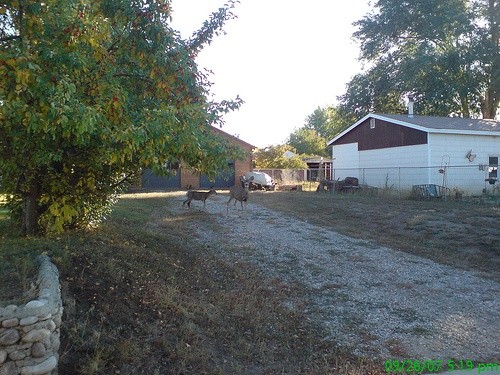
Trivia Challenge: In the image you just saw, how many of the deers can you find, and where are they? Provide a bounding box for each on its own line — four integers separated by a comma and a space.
182, 185, 218, 208
226, 175, 254, 217
